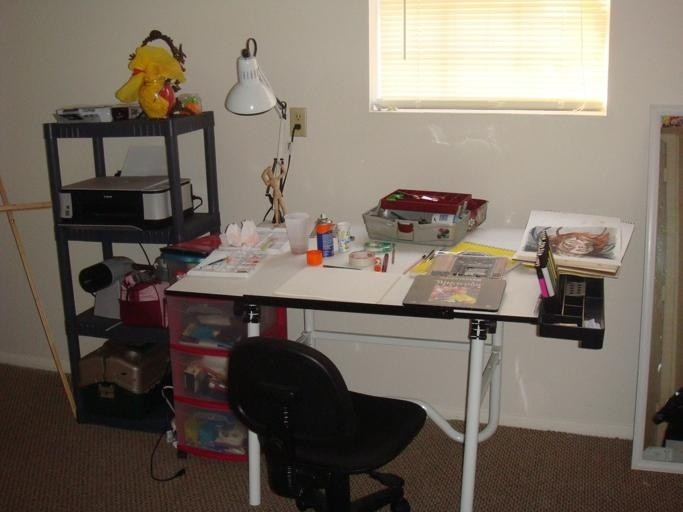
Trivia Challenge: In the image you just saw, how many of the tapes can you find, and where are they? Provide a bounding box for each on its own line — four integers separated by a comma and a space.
349, 250, 375, 268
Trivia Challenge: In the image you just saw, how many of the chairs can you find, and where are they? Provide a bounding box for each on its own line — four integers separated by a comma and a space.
228, 337, 426, 512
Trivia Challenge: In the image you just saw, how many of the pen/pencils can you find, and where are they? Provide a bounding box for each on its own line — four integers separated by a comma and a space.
199, 256, 229, 269
322, 264, 362, 272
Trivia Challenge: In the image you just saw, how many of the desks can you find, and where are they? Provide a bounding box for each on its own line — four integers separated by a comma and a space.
164, 220, 553, 511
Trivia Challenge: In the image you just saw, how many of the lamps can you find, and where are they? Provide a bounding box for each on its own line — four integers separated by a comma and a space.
222, 36, 288, 225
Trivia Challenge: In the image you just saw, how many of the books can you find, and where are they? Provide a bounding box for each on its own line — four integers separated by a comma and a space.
188, 250, 272, 277
514, 210, 634, 279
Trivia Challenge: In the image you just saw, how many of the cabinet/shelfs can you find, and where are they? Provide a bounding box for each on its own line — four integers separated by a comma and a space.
44, 108, 222, 434
166, 295, 288, 462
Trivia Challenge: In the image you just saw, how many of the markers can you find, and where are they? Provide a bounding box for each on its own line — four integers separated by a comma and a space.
375, 256, 381, 271
382, 254, 389, 272
534, 231, 557, 299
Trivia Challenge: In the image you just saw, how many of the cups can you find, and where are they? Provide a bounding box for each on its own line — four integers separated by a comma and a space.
285, 212, 310, 254
336, 222, 351, 253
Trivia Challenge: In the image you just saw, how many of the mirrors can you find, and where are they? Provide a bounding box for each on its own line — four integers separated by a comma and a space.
632, 107, 682, 477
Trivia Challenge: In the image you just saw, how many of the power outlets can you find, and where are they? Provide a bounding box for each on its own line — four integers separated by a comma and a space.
288, 106, 308, 139
